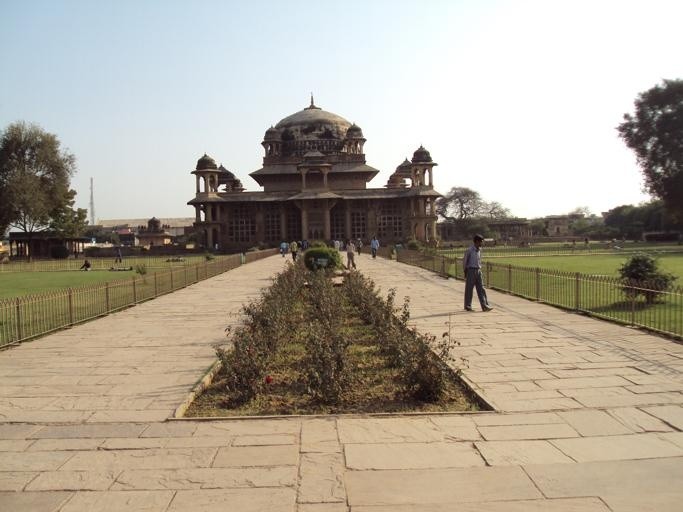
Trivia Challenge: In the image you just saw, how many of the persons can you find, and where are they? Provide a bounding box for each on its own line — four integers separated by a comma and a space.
371, 236, 379, 258
463, 233, 493, 312
346, 241, 356, 270
332, 238, 363, 255
80, 260, 92, 271
281, 239, 309, 262
115, 246, 122, 263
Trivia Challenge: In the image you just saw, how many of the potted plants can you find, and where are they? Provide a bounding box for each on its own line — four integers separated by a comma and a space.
305, 247, 343, 271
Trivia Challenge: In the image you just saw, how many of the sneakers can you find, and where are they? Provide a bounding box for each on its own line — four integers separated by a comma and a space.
467, 305, 494, 311
